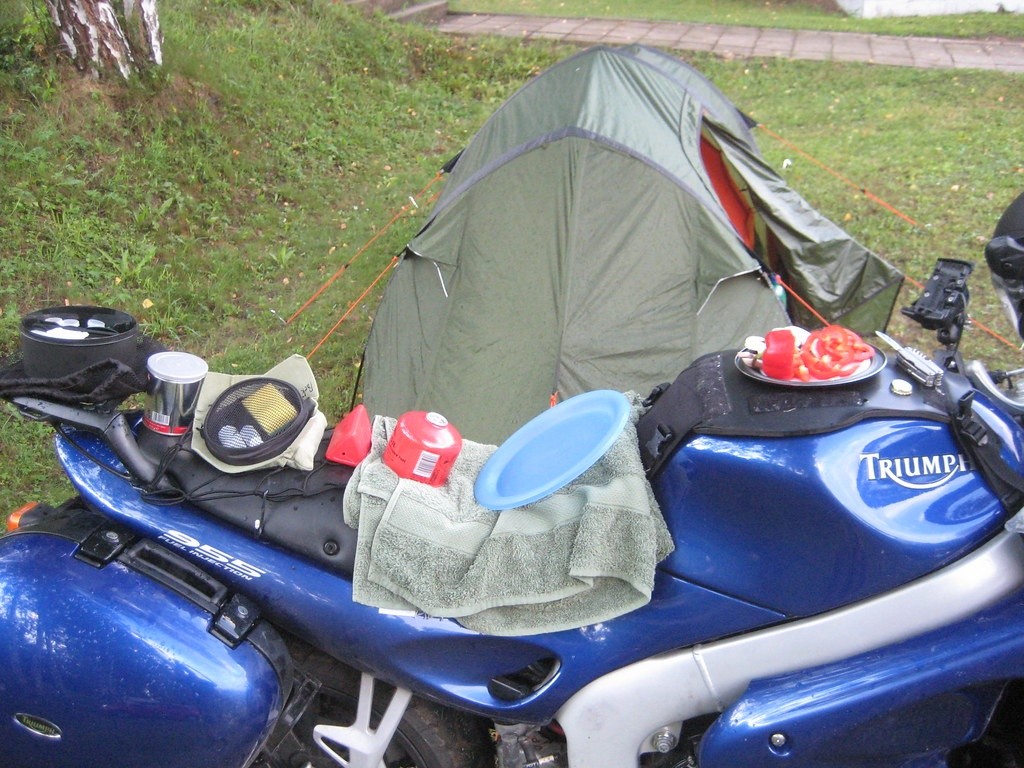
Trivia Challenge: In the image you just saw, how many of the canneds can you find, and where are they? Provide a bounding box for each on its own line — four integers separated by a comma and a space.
142, 352, 209, 436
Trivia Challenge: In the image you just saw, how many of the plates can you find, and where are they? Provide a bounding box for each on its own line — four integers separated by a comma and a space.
733, 343, 888, 387
473, 390, 630, 509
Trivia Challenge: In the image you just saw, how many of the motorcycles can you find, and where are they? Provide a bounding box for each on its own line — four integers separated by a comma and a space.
0, 302, 1024, 768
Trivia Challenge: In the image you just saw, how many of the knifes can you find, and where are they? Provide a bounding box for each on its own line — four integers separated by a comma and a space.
875, 330, 943, 387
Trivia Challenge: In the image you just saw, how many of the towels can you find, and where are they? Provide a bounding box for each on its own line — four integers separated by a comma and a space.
342, 388, 677, 638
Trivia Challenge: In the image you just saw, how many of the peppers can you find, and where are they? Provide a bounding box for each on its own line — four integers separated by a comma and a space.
750, 324, 875, 383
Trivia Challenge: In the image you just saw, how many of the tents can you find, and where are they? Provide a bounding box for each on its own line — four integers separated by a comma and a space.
361, 42, 905, 446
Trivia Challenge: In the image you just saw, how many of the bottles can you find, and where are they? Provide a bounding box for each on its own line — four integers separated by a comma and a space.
384, 411, 463, 487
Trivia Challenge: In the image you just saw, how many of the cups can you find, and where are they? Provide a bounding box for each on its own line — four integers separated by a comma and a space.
141, 350, 209, 437
